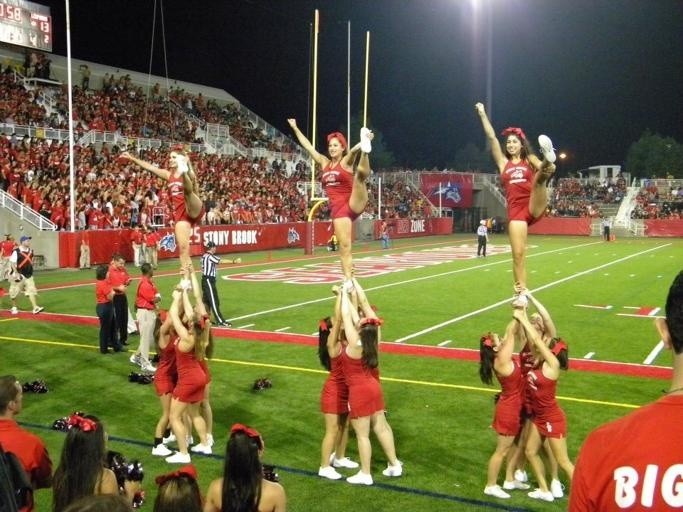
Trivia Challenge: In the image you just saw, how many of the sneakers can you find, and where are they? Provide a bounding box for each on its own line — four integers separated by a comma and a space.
152, 430, 214, 463
484, 469, 563, 502
176, 155, 188, 172
360, 127, 372, 153
101, 340, 129, 352
179, 279, 193, 289
218, 320, 232, 327
318, 453, 401, 485
538, 134, 557, 162
341, 279, 353, 291
11, 307, 18, 314
129, 354, 159, 373
512, 293, 528, 307
32, 305, 44, 314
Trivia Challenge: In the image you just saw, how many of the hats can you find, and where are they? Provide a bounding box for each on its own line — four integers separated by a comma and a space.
20, 235, 32, 242
206, 241, 216, 248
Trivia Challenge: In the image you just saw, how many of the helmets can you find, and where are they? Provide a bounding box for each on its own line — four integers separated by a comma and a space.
480, 220, 487, 225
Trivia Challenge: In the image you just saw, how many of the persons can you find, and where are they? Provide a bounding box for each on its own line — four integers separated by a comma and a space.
0, 53, 330, 226
377, 221, 391, 249
95, 255, 161, 372
479, 281, 576, 501
2, 233, 44, 315
474, 102, 555, 308
151, 264, 214, 464
319, 262, 402, 485
80, 225, 91, 270
288, 118, 374, 292
546, 170, 626, 218
120, 143, 205, 291
200, 242, 241, 327
568, 271, 683, 511
1, 373, 287, 511
600, 217, 615, 241
631, 179, 682, 219
364, 179, 434, 221
